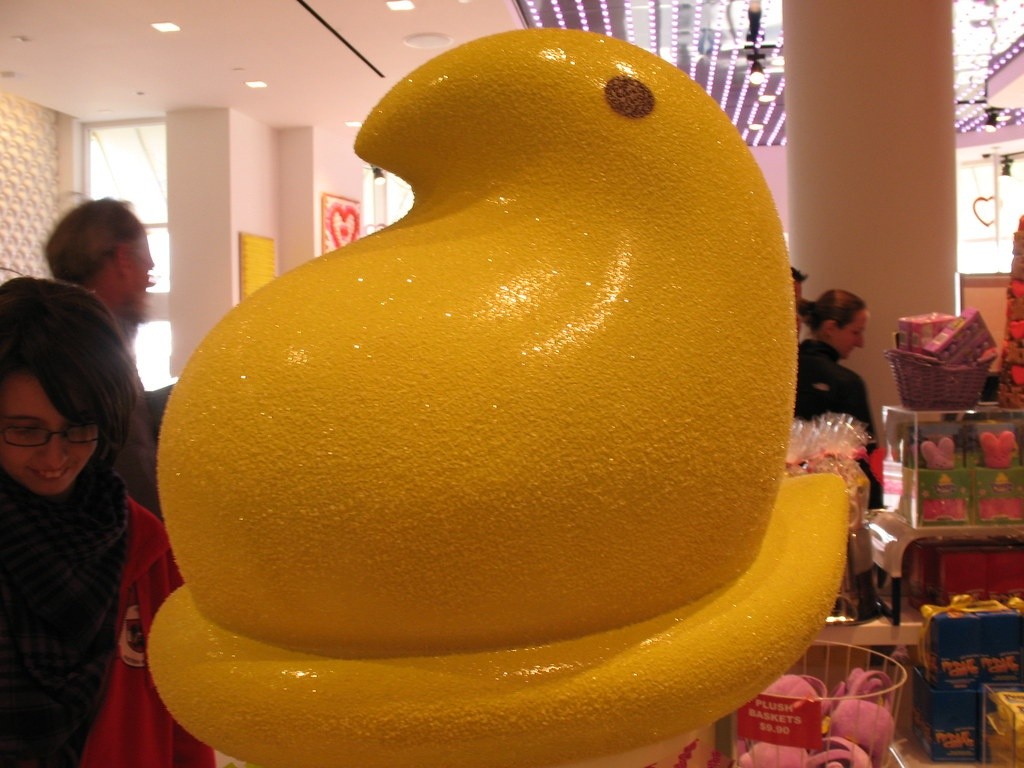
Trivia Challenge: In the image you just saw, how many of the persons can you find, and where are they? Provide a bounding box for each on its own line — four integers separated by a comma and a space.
45, 199, 174, 515
0, 276, 216, 768
796, 289, 883, 509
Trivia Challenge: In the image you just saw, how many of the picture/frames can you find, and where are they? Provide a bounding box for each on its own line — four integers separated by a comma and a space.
319, 193, 361, 257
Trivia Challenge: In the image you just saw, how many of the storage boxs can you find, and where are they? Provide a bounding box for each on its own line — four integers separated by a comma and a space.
882, 307, 1024, 768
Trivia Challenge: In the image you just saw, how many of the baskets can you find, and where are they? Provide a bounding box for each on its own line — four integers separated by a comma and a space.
884, 346, 994, 410
734, 638, 907, 767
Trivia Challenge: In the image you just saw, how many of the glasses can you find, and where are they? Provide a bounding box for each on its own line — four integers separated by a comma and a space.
0, 417, 99, 447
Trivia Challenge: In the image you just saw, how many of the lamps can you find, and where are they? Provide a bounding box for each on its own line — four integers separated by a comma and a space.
747, 55, 767, 85
1001, 159, 1013, 178
984, 110, 998, 133
371, 166, 384, 184
747, 0, 762, 36
